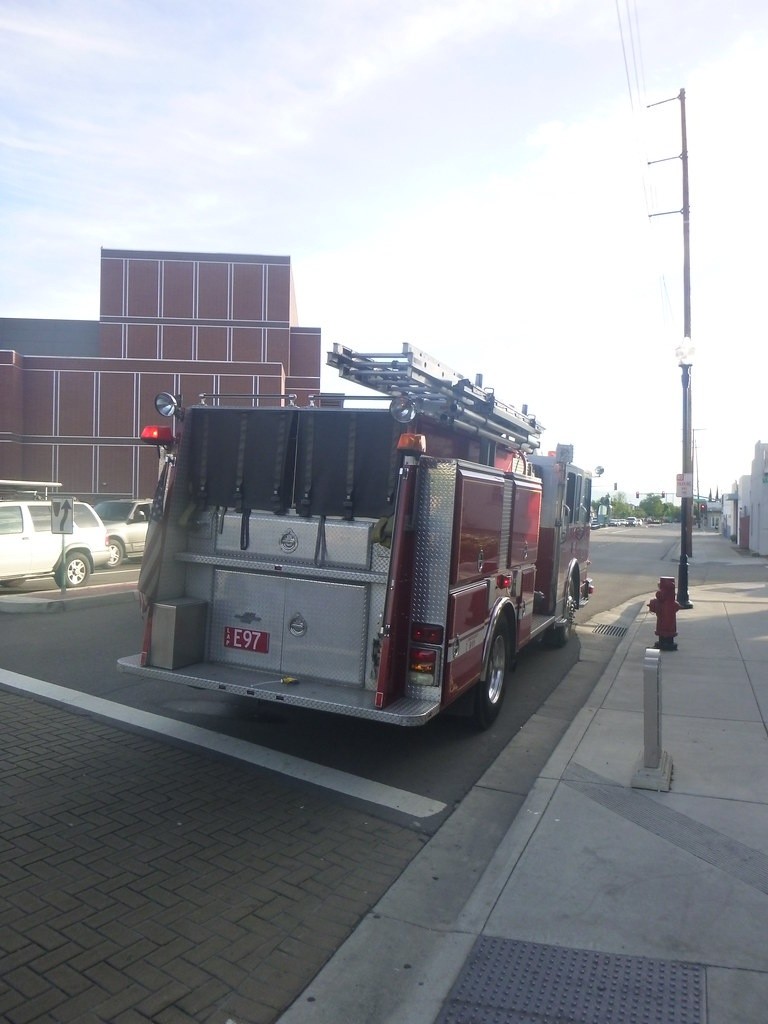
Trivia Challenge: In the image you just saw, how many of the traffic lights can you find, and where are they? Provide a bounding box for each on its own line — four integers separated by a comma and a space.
662, 491, 665, 498
636, 491, 639, 498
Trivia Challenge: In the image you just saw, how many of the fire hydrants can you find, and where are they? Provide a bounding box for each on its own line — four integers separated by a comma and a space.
645, 577, 683, 652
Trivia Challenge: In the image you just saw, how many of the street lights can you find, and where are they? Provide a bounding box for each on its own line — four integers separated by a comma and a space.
675, 333, 696, 610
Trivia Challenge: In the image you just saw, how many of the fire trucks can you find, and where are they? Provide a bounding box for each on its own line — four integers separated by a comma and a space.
136, 340, 594, 733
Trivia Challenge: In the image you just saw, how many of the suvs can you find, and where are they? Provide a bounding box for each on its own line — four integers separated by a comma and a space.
0, 479, 112, 589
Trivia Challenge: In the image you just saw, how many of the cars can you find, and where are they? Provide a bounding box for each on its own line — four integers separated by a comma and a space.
91, 498, 152, 571
591, 517, 643, 530
